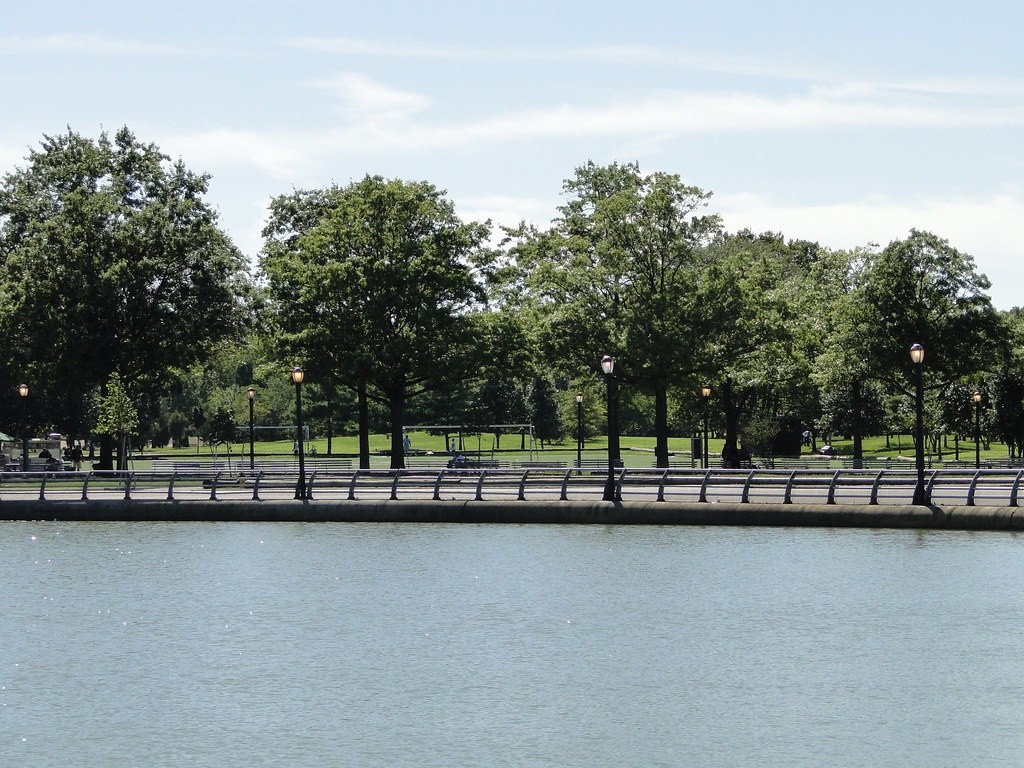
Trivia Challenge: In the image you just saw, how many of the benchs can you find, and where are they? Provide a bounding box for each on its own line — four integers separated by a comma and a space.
152, 460, 225, 482
842, 457, 1024, 469
708, 460, 831, 469
652, 461, 698, 469
29, 463, 71, 476
511, 461, 568, 476
573, 459, 624, 475
235, 460, 510, 480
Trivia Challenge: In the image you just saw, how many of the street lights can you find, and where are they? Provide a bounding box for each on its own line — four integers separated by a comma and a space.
910, 344, 926, 505
576, 393, 584, 476
245, 388, 255, 476
19, 384, 29, 471
601, 355, 614, 501
702, 385, 711, 468
973, 392, 981, 467
290, 368, 305, 499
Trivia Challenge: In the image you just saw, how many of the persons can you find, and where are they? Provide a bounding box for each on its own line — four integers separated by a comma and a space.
403, 435, 411, 455
70, 445, 84, 471
451, 439, 456, 452
292, 438, 299, 456
39, 448, 59, 470
310, 445, 317, 458
